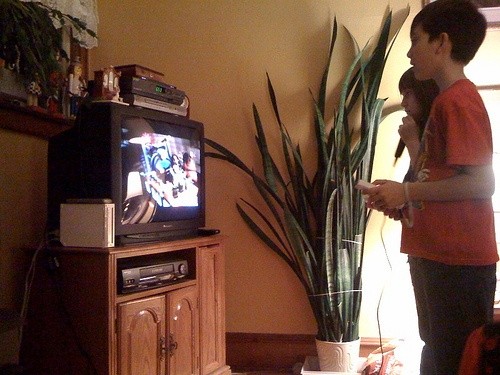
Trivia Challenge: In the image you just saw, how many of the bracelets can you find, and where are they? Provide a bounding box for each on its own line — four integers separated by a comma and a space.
403, 182, 411, 203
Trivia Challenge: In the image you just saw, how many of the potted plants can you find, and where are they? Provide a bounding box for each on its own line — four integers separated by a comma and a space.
204, 6, 408, 375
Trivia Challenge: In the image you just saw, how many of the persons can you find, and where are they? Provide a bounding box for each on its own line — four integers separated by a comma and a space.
135, 131, 197, 191
398, 66, 440, 184
362, 0, 499, 375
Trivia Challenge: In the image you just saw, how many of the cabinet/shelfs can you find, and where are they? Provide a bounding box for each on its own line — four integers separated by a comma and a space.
0, 1, 102, 137
18, 230, 232, 375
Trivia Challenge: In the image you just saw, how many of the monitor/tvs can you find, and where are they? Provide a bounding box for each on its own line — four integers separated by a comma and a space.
48, 101, 206, 246
